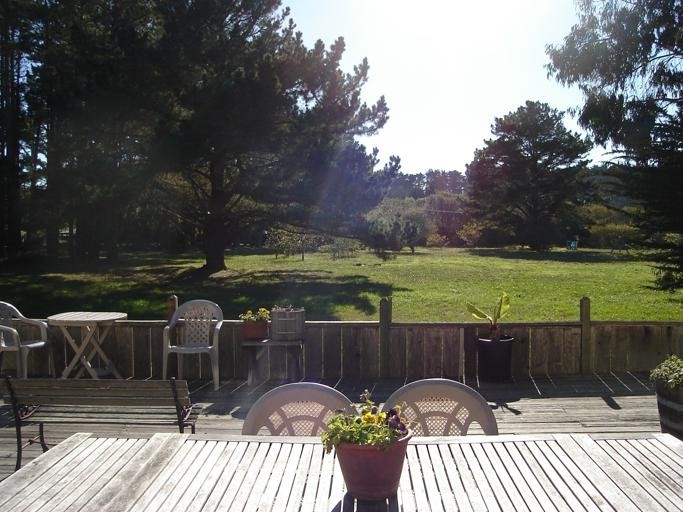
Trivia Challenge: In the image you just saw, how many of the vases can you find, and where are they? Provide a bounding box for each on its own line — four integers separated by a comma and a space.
330, 428, 413, 504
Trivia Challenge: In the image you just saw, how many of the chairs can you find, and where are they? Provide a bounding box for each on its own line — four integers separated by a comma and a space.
240, 381, 359, 440
374, 377, 500, 440
0, 299, 56, 381
160, 298, 224, 392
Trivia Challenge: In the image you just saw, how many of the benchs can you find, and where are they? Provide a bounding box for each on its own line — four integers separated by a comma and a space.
0, 373, 204, 477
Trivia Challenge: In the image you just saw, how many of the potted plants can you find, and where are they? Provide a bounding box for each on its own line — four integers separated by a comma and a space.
464, 289, 515, 384
237, 306, 271, 343
270, 305, 305, 342
645, 353, 683, 442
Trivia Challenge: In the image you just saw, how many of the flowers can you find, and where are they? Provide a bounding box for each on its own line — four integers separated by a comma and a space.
317, 386, 416, 461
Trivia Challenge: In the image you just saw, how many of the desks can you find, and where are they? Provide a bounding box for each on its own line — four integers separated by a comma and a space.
240, 338, 305, 387
45, 310, 129, 380
0, 429, 683, 512
